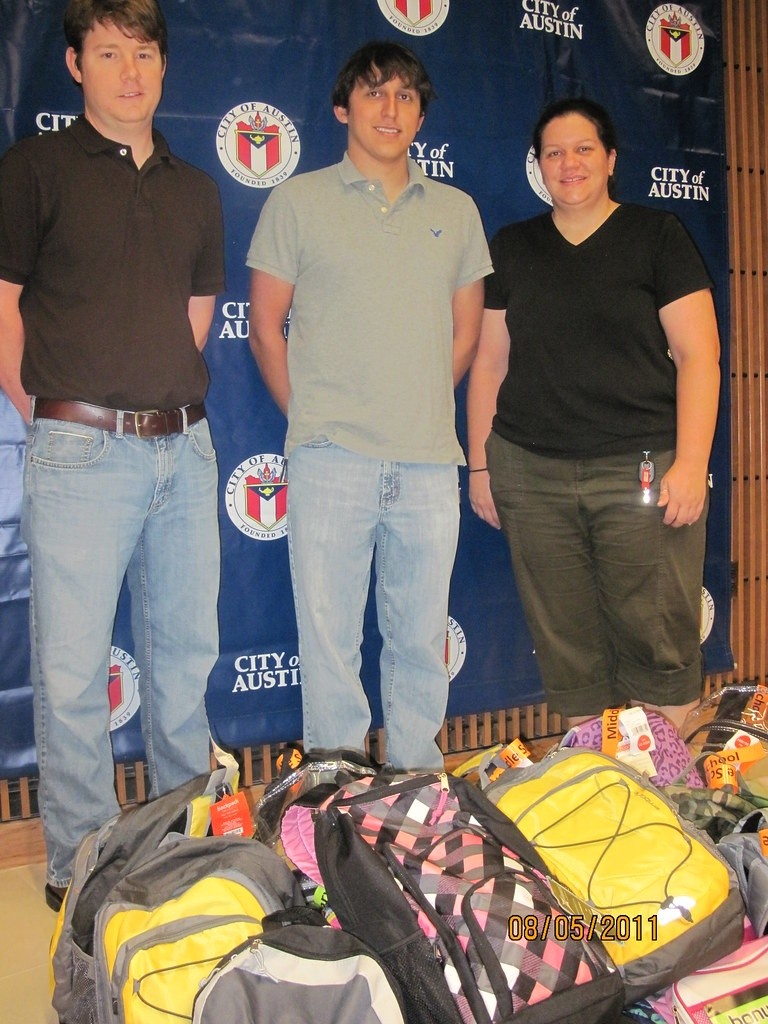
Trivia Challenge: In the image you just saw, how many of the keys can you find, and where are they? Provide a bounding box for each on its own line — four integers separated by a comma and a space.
638, 461, 654, 503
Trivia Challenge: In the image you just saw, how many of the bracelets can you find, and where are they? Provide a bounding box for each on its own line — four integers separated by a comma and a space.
469, 468, 488, 472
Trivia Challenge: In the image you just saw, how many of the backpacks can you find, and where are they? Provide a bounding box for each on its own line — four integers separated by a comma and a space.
47, 681, 768, 1024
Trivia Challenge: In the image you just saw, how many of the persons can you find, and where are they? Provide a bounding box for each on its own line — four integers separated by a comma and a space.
245, 40, 496, 772
0, 0, 225, 906
469, 98, 720, 737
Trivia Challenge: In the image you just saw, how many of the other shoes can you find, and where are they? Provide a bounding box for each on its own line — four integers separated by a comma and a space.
44, 884, 66, 908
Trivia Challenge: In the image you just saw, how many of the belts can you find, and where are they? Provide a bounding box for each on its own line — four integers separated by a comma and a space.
33, 399, 206, 439
